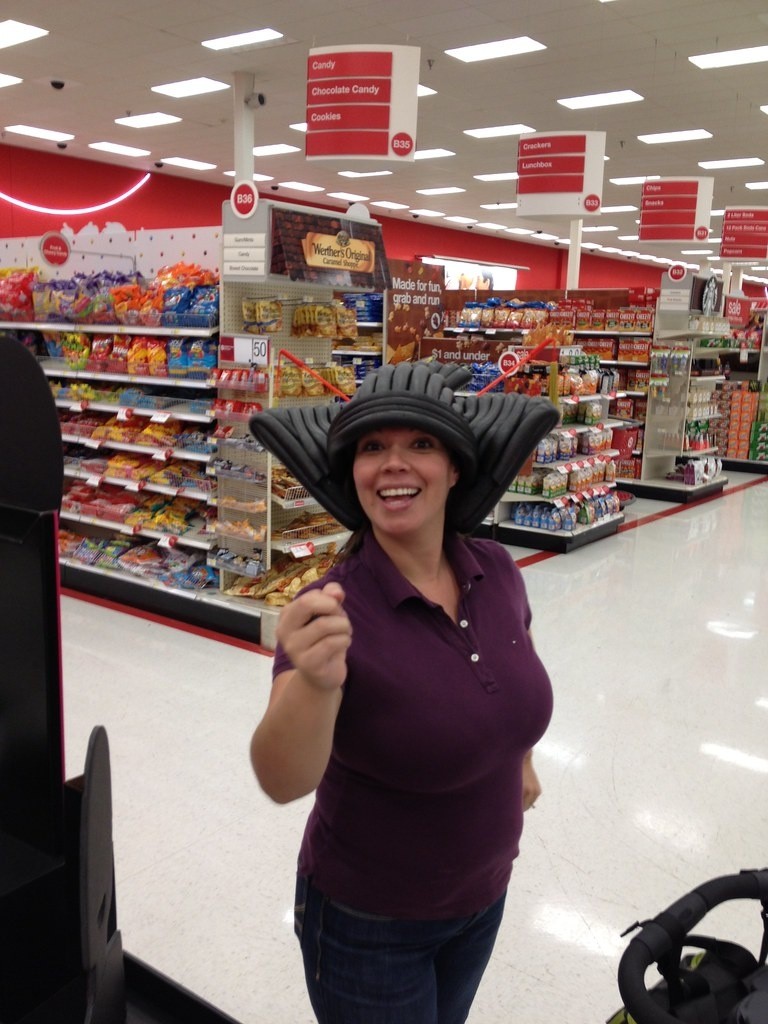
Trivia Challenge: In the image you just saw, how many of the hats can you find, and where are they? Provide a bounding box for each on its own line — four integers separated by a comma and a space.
252, 338, 559, 533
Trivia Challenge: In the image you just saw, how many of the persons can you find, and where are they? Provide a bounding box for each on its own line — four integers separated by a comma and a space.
251, 360, 555, 1024
456, 271, 494, 291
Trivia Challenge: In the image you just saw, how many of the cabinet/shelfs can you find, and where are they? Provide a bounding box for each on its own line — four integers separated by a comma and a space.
0, 200, 768, 648
